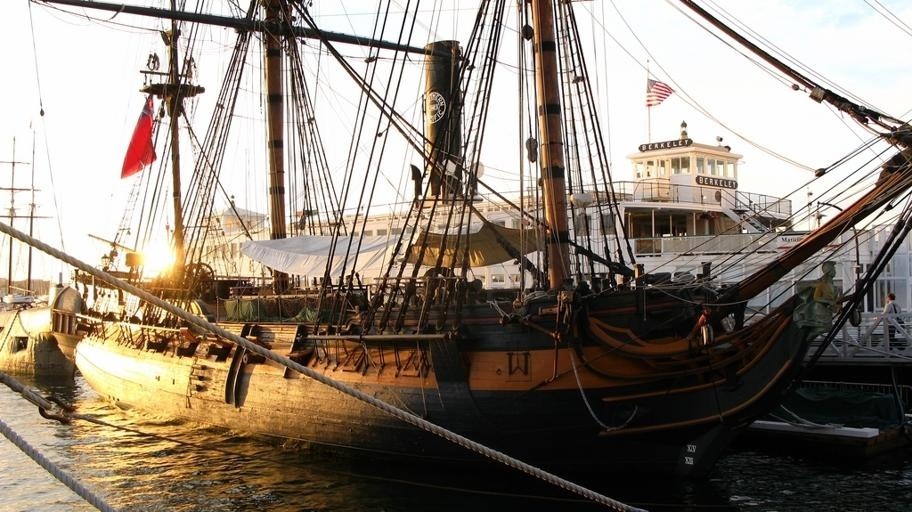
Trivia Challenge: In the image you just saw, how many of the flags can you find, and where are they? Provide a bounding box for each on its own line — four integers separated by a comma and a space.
119, 94, 156, 177
646, 79, 673, 106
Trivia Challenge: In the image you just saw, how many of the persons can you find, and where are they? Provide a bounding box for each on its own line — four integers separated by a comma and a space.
794, 261, 841, 339
881, 293, 901, 351
